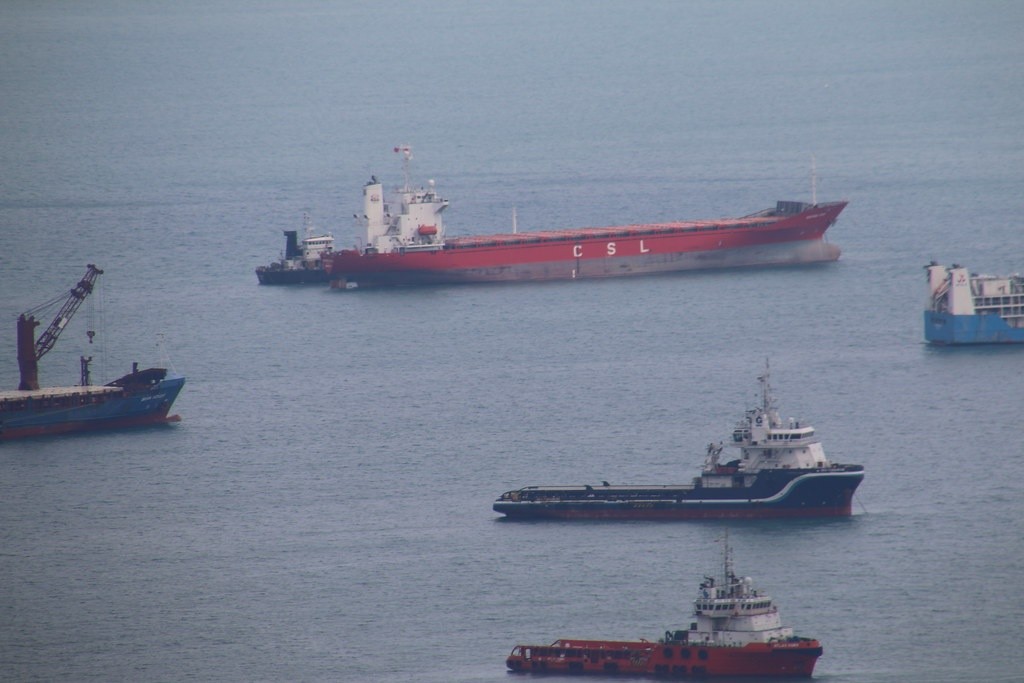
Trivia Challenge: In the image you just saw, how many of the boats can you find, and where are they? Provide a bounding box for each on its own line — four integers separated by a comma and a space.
320, 140, 849, 289
493, 357, 865, 518
255, 213, 341, 285
0, 263, 186, 443
506, 527, 823, 678
923, 260, 1024, 343
